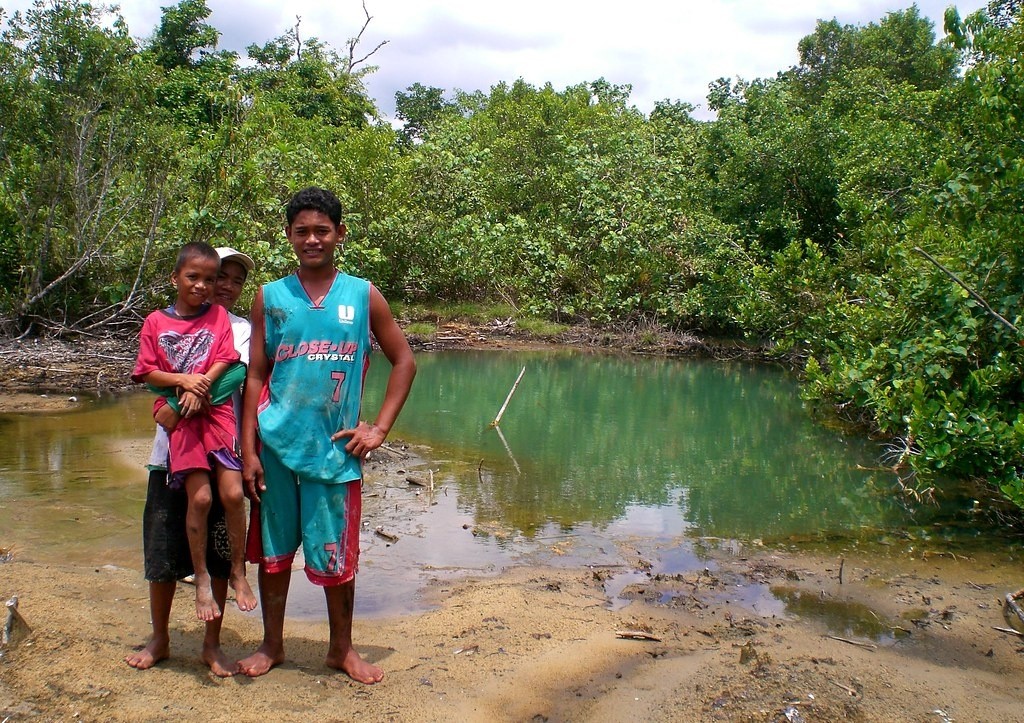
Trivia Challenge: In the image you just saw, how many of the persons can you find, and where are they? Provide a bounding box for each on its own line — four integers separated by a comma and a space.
239, 188, 417, 683
126, 247, 251, 676
131, 242, 258, 621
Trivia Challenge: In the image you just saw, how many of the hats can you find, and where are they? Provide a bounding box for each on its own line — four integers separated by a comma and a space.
214, 246, 255, 271
